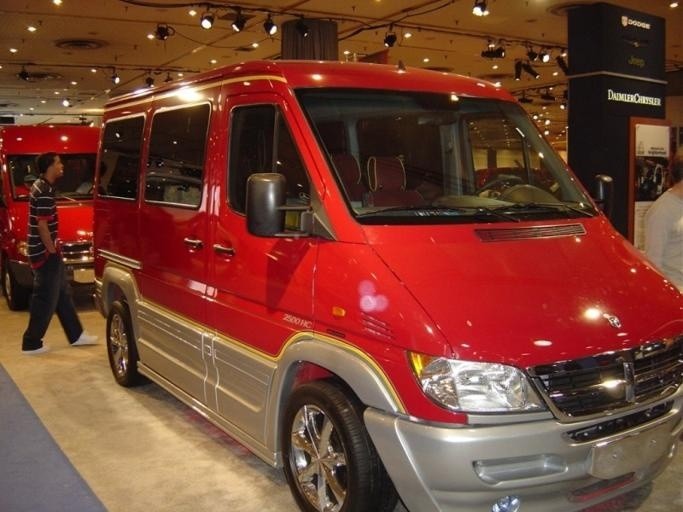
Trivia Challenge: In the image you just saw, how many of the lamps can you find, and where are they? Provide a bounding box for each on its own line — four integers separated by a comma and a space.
381, 23, 398, 48
471, 0, 488, 18
478, 39, 568, 107
198, 5, 279, 37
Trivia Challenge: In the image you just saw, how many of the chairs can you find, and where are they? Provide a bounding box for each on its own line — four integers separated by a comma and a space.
330, 150, 422, 211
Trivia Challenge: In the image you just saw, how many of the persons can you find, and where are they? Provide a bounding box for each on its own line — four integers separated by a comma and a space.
21, 152, 98, 353
642, 154, 683, 291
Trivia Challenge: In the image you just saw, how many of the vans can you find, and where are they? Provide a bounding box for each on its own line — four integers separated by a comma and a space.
90, 60, 681, 511
0, 123, 107, 312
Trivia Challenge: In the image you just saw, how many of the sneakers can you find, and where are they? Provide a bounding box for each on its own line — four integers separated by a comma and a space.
72, 331, 101, 345
22, 348, 46, 355
